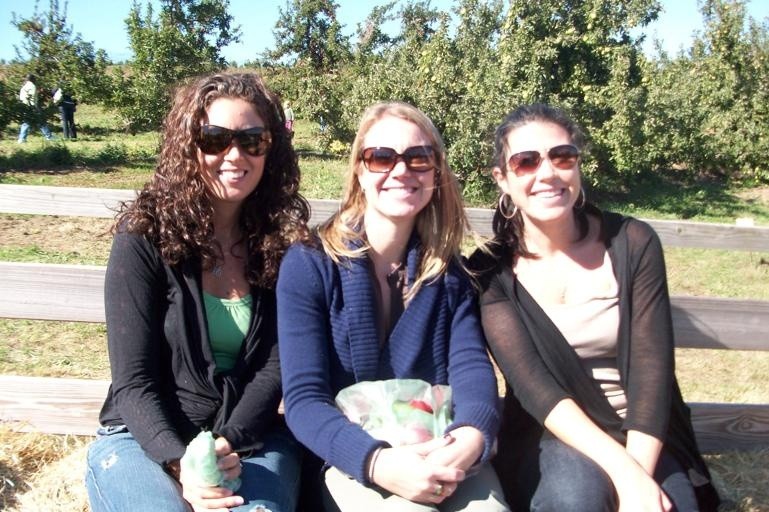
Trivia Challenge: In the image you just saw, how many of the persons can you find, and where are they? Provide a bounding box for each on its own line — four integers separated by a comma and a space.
16, 74, 59, 144
274, 98, 516, 511
82, 71, 315, 512
469, 103, 721, 511
49, 74, 78, 142
281, 101, 294, 132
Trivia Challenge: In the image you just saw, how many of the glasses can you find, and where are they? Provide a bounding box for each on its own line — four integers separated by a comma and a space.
358, 144, 439, 174
192, 123, 274, 158
506, 143, 579, 180
